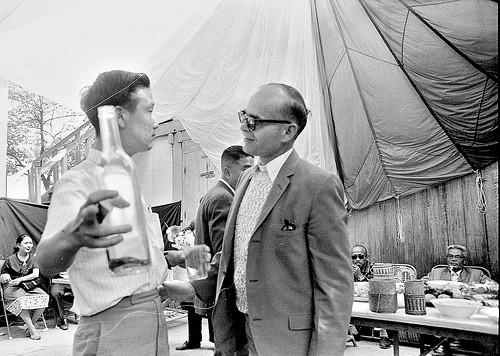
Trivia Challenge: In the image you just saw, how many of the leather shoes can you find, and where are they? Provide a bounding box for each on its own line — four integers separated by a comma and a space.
379, 336, 390, 349
175, 341, 201, 349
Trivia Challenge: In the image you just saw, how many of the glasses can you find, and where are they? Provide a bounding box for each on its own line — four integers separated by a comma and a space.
238, 110, 294, 131
280, 217, 296, 231
351, 254, 366, 260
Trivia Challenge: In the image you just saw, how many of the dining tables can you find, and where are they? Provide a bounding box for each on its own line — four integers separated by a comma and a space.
351, 293, 499, 356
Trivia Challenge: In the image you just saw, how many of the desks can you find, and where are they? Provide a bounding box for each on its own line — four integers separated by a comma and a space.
52, 278, 79, 325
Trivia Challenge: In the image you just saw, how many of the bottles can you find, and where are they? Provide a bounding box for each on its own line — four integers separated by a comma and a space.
96, 105, 151, 274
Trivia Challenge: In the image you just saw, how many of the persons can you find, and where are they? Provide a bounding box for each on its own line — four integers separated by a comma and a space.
194, 145, 254, 262
175, 197, 214, 350
158, 82, 354, 356
422, 245, 498, 286
0, 234, 49, 339
348, 245, 391, 349
32, 70, 211, 356
50, 274, 80, 330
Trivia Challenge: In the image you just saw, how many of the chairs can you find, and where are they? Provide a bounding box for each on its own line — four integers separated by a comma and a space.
371, 263, 417, 283
0, 259, 48, 340
431, 264, 491, 286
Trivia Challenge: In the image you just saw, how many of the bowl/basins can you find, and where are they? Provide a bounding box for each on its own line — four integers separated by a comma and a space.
432, 298, 483, 318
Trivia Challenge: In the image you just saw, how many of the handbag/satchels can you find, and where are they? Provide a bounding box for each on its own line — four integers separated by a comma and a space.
20, 277, 43, 292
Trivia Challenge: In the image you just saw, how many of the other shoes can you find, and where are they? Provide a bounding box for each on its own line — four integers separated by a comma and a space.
29, 332, 41, 340
26, 328, 30, 336
58, 321, 69, 330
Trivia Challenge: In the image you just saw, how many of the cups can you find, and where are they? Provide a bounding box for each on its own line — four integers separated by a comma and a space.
184, 246, 208, 280
